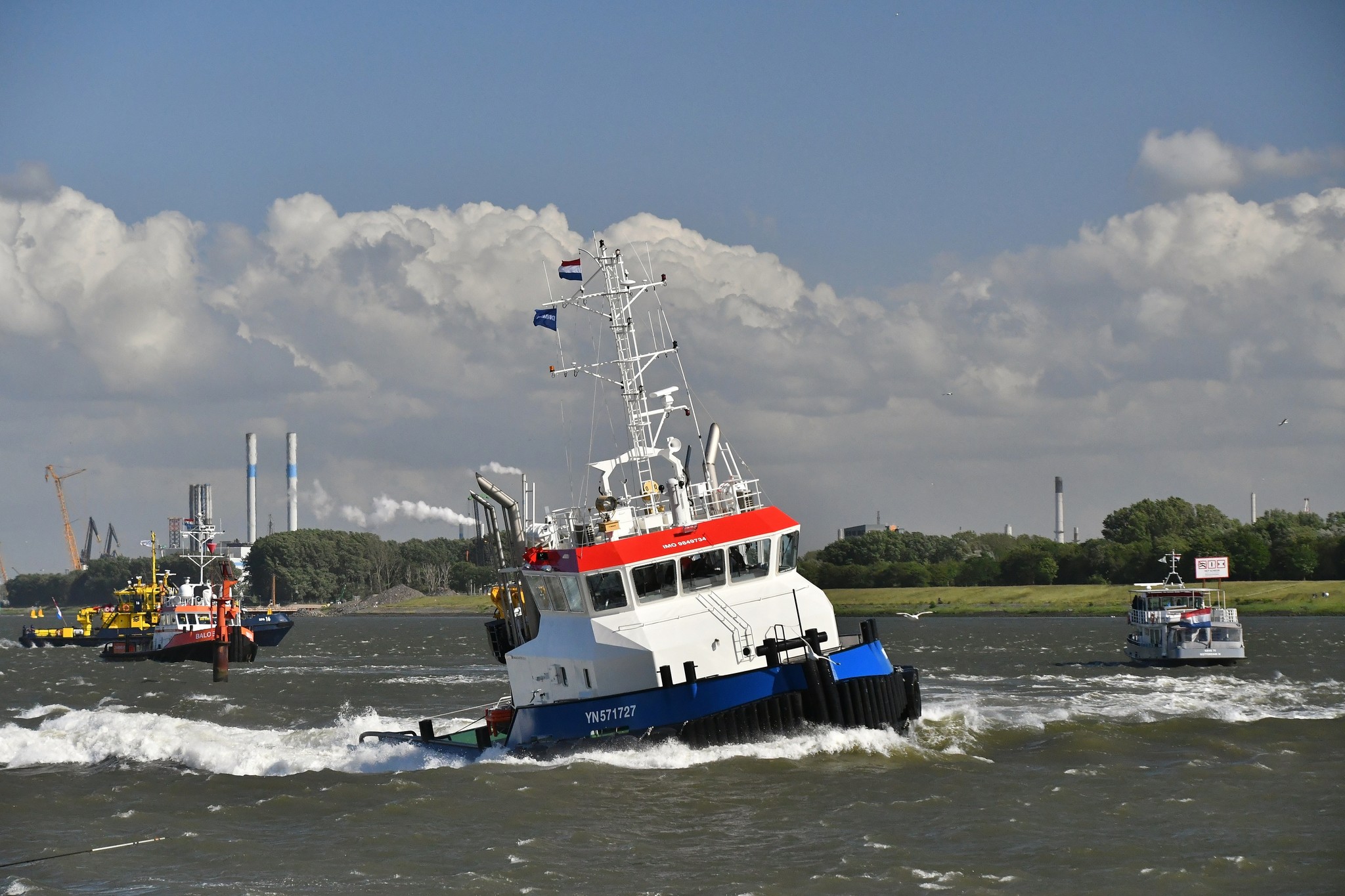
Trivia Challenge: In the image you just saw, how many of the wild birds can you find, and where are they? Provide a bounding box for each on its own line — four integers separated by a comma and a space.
1261, 478, 1265, 481
942, 392, 953, 396
895, 611, 934, 621
530, 688, 542, 703
931, 483, 934, 485
1278, 417, 1289, 427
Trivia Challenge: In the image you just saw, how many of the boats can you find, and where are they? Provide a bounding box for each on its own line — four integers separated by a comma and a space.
199, 612, 295, 646
1123, 548, 1248, 666
99, 543, 257, 664
18, 530, 179, 648
360, 232, 924, 766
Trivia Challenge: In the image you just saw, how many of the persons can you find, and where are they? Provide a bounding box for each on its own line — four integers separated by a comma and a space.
1176, 599, 1182, 606
180, 618, 185, 624
1131, 595, 1143, 622
665, 547, 746, 585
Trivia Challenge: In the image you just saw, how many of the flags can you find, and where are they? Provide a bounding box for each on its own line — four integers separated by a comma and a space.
558, 259, 582, 280
183, 519, 194, 527
1179, 607, 1212, 627
533, 308, 557, 332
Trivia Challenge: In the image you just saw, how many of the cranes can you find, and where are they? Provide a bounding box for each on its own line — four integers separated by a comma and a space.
103, 523, 121, 556
44, 465, 88, 571
80, 516, 101, 567
0, 557, 8, 582
